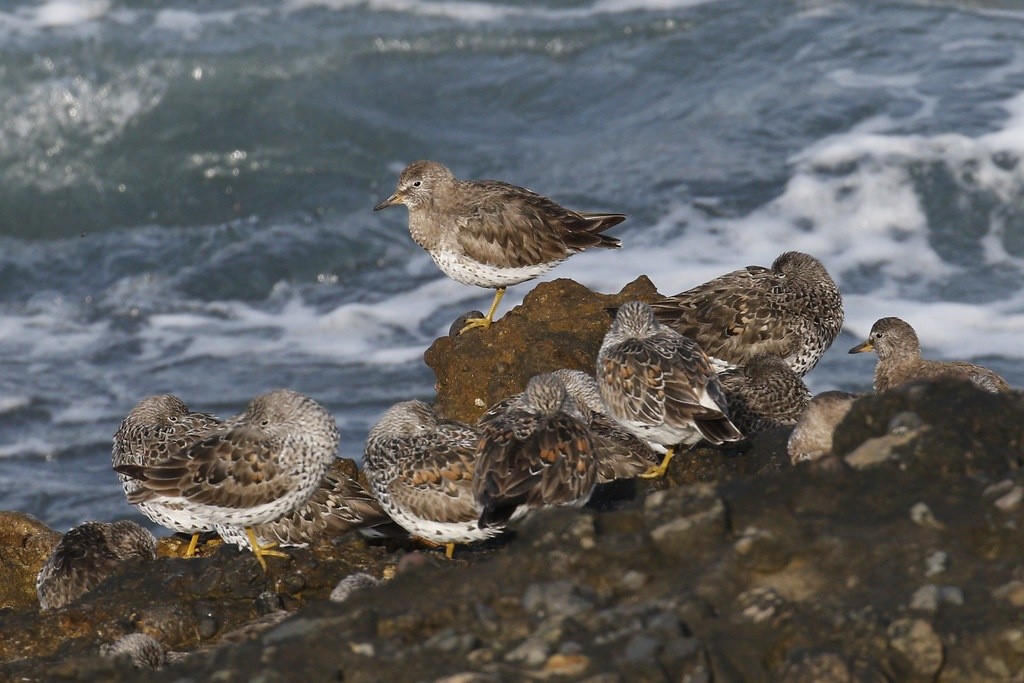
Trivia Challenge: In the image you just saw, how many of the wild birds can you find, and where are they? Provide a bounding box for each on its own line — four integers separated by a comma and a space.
330, 572, 379, 604
648, 250, 844, 378
112, 389, 340, 569
107, 633, 191, 674
597, 300, 742, 480
363, 399, 506, 582
373, 160, 630, 339
716, 353, 814, 432
786, 390, 867, 466
36, 520, 159, 609
472, 368, 660, 530
847, 317, 1013, 396
